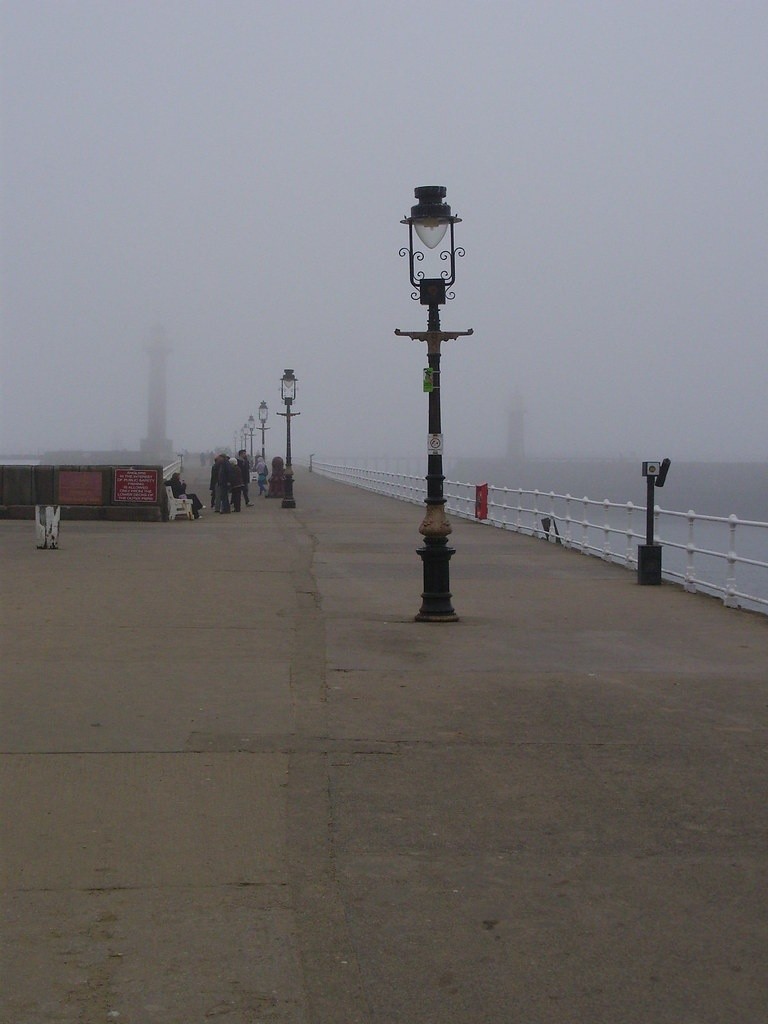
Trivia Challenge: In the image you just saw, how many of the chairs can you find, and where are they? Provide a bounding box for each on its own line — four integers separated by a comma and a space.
249, 471, 258, 482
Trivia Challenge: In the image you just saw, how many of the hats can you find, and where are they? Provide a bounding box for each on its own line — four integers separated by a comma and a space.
229, 457, 238, 465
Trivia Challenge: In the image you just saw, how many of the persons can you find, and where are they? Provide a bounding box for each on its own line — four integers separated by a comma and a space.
256, 457, 268, 496
171, 473, 206, 519
209, 450, 254, 514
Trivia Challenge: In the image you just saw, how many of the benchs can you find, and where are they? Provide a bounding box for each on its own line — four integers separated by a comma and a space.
165, 485, 196, 522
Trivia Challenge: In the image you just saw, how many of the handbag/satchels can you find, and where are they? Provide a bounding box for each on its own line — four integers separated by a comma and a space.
264, 465, 268, 476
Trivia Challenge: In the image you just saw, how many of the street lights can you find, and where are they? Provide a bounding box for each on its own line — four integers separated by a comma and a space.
239, 428, 243, 450
243, 424, 249, 451
276, 369, 300, 508
247, 415, 255, 458
394, 186, 474, 623
256, 401, 271, 460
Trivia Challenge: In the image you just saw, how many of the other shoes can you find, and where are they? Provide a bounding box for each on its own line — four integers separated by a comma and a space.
201, 506, 206, 509
247, 502, 254, 507
198, 515, 202, 518
